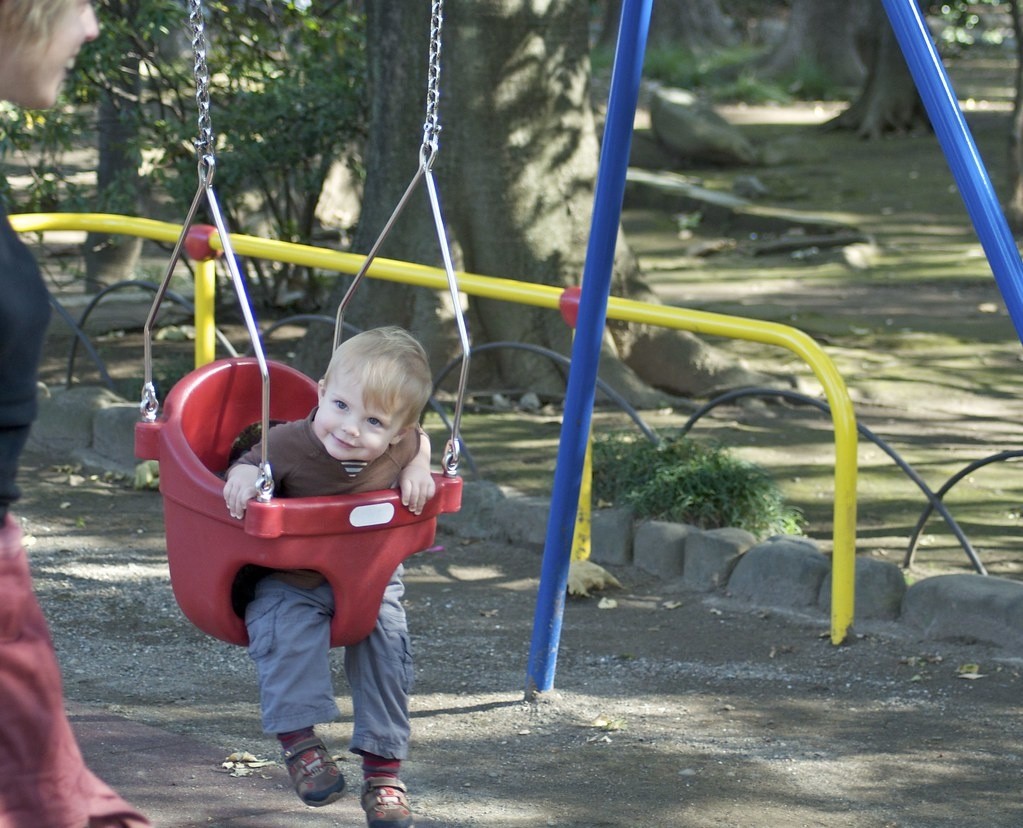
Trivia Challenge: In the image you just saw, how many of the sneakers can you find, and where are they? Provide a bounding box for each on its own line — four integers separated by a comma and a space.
281, 736, 347, 807
361, 777, 415, 828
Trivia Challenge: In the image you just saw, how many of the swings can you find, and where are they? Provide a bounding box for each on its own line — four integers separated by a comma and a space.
128, 1, 470, 651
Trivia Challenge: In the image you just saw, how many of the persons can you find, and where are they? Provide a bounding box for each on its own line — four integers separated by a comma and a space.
0, 0, 152, 828
223, 325, 436, 828
